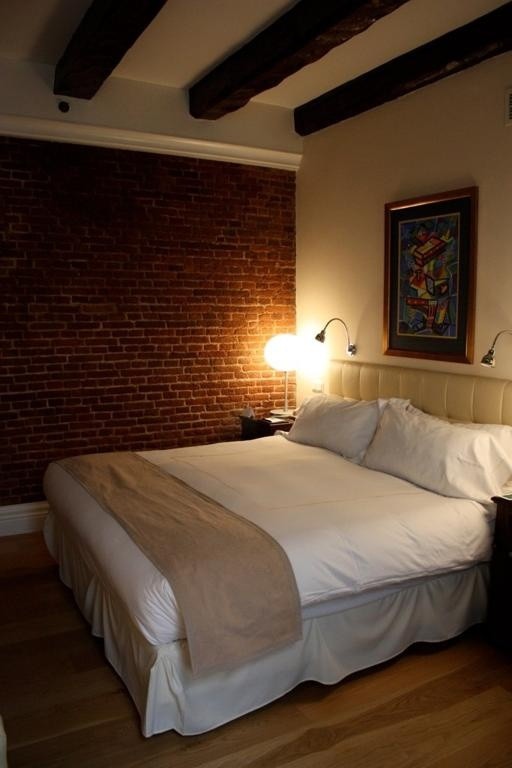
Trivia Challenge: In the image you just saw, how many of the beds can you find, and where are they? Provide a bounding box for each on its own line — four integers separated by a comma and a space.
36, 359, 512, 738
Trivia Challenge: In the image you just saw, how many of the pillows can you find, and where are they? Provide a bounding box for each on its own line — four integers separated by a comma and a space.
450, 418, 511, 489
356, 399, 510, 506
281, 387, 411, 465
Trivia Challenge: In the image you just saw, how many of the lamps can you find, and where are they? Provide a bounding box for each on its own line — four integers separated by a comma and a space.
479, 327, 512, 370
313, 318, 357, 358
261, 328, 304, 417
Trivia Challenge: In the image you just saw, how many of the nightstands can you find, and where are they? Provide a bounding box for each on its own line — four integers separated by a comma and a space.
478, 491, 512, 670
236, 408, 296, 439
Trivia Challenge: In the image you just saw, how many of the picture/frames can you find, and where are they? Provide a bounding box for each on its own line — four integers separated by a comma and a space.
380, 183, 480, 368
